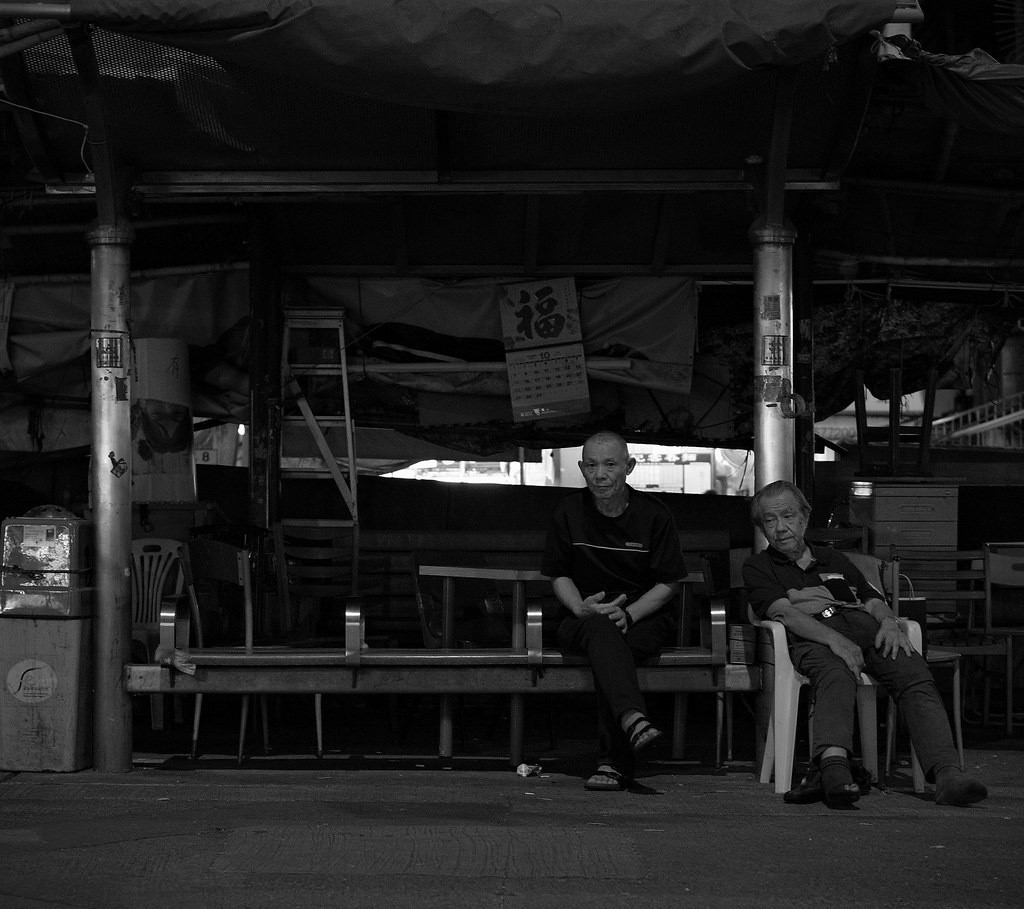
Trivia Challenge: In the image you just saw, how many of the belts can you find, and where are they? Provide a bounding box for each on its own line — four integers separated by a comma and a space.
814, 604, 839, 621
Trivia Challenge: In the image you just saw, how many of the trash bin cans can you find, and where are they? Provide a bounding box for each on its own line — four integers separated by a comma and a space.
0, 517, 93, 773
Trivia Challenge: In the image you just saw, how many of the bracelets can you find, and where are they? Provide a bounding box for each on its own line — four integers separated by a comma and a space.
620, 606, 633, 627
878, 614, 899, 625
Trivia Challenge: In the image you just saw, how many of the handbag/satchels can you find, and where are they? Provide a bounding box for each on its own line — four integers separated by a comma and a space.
897, 574, 928, 657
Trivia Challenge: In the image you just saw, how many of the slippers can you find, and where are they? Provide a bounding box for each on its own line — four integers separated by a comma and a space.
584, 764, 630, 790
626, 718, 662, 751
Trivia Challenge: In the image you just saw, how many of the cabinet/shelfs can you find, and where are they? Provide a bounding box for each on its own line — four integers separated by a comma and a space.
869, 484, 957, 622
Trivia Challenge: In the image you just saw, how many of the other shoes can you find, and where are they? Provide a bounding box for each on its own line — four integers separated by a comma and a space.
782, 763, 871, 802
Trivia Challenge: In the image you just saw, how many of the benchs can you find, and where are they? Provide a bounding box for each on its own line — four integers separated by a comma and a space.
126, 603, 727, 769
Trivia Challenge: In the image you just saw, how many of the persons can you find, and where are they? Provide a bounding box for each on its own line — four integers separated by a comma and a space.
742, 480, 988, 809
539, 432, 690, 788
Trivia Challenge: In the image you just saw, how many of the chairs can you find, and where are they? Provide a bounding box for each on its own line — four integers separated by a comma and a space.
748, 539, 1024, 794
130, 539, 185, 730
